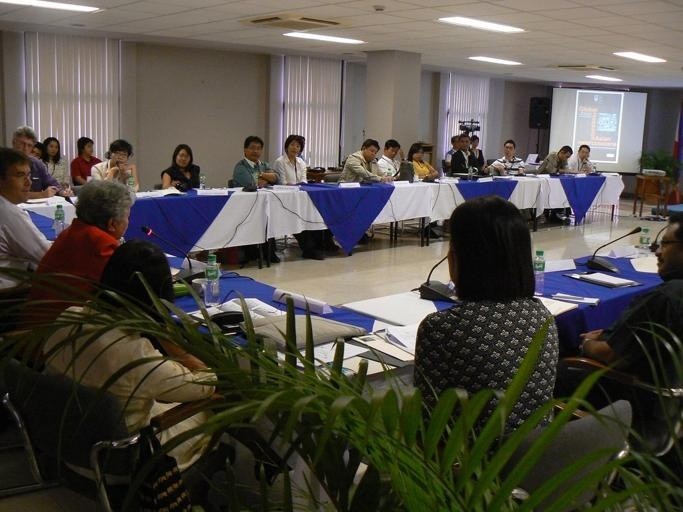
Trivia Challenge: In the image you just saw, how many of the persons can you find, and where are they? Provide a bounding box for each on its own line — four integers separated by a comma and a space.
0, 149, 51, 291
21, 179, 132, 362
273, 135, 338, 260
12, 125, 139, 199
40, 239, 217, 474
579, 210, 683, 437
232, 136, 280, 263
161, 143, 201, 192
341, 135, 590, 242
413, 196, 559, 456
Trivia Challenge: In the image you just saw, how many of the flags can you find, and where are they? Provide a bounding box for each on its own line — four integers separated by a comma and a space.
672, 110, 683, 190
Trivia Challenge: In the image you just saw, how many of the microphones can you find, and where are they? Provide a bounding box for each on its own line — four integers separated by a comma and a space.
587, 226, 642, 274
64, 195, 76, 207
360, 162, 372, 186
115, 160, 121, 170
240, 159, 256, 191
140, 223, 205, 284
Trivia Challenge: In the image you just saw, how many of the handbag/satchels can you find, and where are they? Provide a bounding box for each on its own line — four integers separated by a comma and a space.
131, 454, 194, 512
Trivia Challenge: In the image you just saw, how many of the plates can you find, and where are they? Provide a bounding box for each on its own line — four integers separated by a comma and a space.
172, 281, 194, 298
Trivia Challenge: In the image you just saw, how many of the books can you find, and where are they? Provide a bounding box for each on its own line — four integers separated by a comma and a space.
349, 331, 413, 369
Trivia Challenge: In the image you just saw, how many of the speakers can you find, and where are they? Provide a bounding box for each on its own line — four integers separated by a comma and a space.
530, 97, 551, 129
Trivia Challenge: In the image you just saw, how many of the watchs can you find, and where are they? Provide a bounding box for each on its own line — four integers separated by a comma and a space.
580, 338, 591, 352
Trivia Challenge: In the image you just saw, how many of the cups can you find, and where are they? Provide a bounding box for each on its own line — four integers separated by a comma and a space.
191, 278, 209, 297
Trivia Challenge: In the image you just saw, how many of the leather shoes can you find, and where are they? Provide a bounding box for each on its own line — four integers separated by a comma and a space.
264, 251, 280, 263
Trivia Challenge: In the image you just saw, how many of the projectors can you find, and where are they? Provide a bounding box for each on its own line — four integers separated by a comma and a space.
643, 169, 666, 177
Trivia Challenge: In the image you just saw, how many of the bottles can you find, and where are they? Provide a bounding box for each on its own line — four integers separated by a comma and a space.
593, 162, 597, 172
533, 249, 546, 296
386, 168, 393, 184
254, 170, 259, 187
638, 228, 651, 264
53, 204, 66, 238
127, 174, 135, 194
203, 254, 220, 308
199, 172, 208, 188
467, 164, 474, 181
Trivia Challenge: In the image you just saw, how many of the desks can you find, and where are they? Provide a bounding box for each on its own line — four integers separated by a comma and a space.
344, 247, 682, 511
0, 166, 626, 269
0, 211, 373, 508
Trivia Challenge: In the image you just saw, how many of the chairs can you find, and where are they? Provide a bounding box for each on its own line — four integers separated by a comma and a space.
632, 176, 670, 219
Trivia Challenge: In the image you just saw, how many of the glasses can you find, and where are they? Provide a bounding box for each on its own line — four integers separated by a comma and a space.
4, 173, 33, 182
110, 150, 128, 158
659, 237, 681, 248
